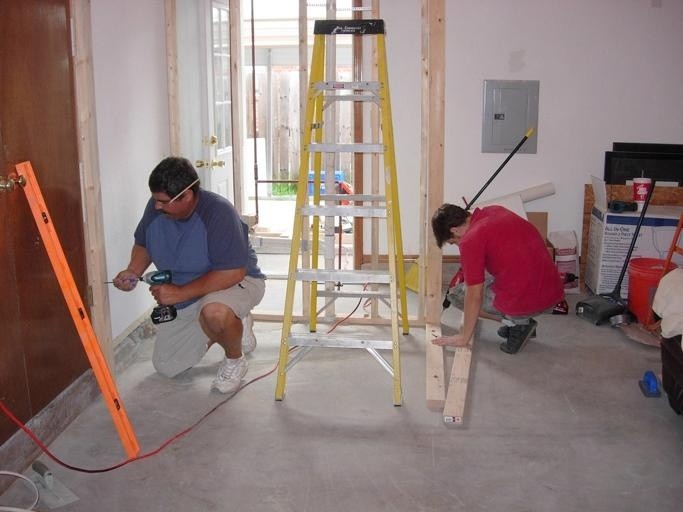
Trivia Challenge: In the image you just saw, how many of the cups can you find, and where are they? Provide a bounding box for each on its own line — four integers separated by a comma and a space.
633, 178, 651, 214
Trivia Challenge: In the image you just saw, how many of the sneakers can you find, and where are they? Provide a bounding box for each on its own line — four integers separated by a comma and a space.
242, 313, 257, 354
498, 317, 537, 354
211, 353, 248, 395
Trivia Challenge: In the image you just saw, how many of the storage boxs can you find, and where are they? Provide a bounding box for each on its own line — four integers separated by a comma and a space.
549, 230, 580, 295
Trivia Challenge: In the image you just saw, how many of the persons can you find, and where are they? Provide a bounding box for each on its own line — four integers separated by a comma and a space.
431, 202, 565, 354
113, 156, 266, 394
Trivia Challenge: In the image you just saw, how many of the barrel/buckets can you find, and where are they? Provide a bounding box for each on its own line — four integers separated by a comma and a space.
626, 257, 679, 329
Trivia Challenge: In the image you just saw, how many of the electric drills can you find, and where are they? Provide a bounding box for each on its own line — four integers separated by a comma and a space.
104, 269, 177, 324
441, 265, 461, 315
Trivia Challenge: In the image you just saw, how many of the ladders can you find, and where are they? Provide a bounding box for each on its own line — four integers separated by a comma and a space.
274, 19, 410, 406
644, 212, 683, 334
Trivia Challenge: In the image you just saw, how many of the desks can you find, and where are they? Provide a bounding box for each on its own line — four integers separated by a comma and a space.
584, 205, 683, 300
